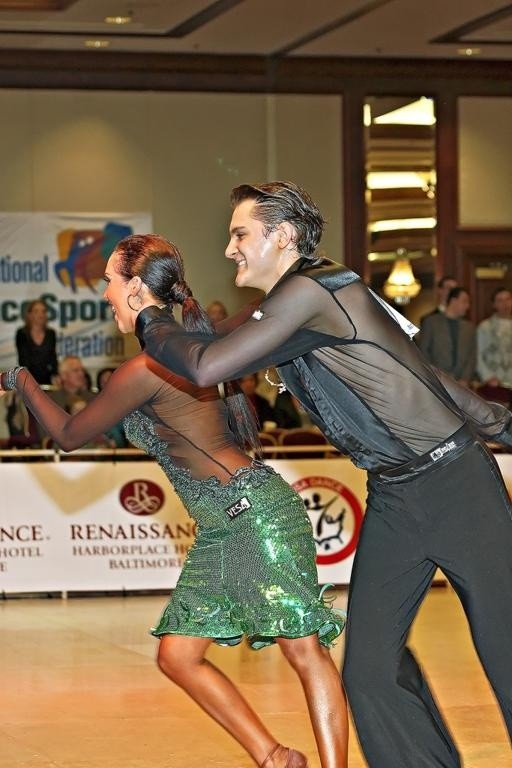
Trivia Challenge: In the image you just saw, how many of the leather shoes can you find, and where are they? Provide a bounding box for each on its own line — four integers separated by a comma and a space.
261, 743, 307, 767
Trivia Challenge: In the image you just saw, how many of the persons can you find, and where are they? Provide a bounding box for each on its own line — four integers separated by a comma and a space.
2, 271, 510, 456
131, 174, 511, 767
0, 232, 351, 767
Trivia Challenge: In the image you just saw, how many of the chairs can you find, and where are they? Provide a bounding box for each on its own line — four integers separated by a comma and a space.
256, 424, 330, 458
